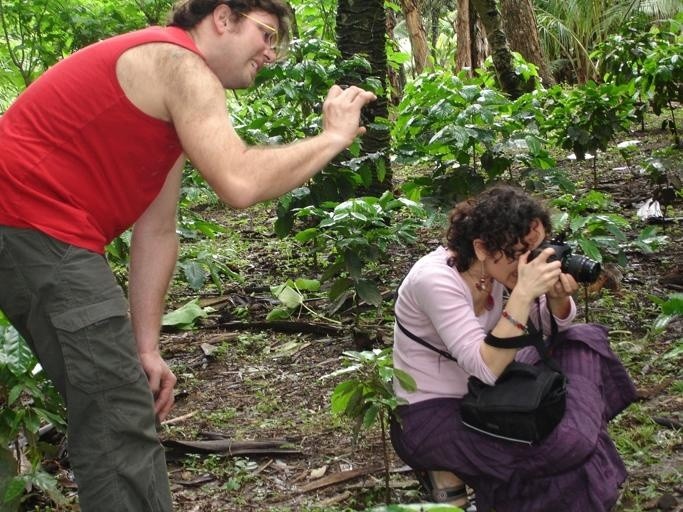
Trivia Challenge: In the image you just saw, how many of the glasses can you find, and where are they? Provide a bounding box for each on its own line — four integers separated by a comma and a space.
240, 12, 282, 59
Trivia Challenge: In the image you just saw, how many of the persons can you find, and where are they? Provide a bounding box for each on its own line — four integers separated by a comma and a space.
390, 189, 637, 512
0, 1, 377, 512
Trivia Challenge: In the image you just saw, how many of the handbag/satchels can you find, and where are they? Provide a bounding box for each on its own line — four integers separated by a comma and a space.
460, 362, 567, 448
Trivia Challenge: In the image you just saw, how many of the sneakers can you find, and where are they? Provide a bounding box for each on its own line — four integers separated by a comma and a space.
415, 470, 477, 512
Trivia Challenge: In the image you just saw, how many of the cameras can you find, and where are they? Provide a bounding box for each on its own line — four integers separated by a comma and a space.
361, 100, 377, 126
528, 241, 600, 283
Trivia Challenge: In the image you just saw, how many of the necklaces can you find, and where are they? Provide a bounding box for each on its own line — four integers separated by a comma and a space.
467, 261, 495, 311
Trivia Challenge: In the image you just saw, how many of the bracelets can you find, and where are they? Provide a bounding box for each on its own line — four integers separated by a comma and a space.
502, 311, 529, 335
